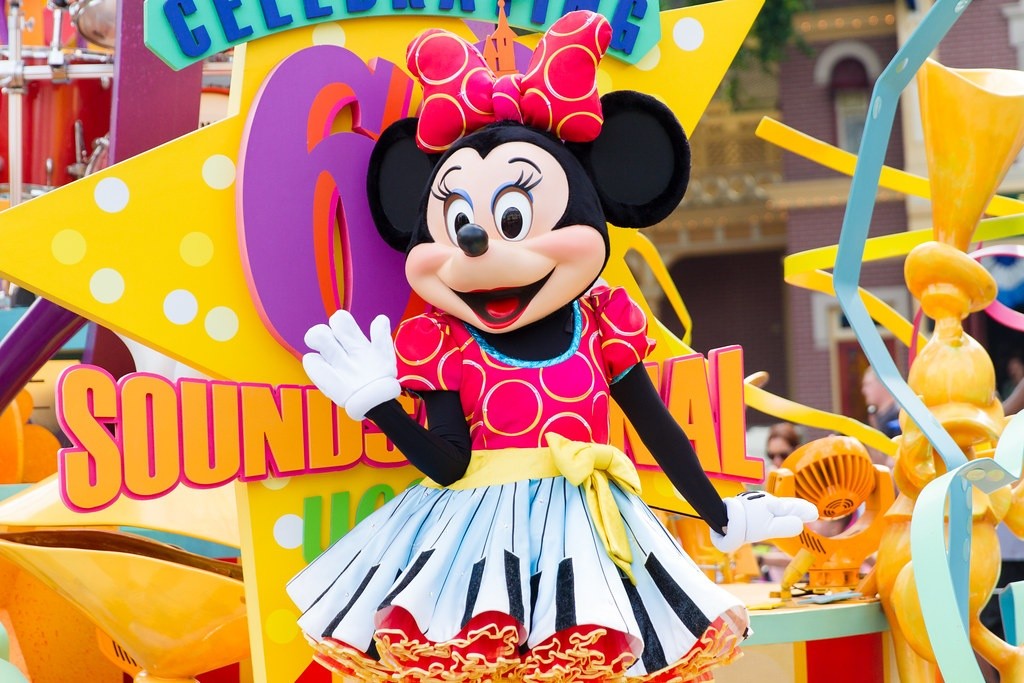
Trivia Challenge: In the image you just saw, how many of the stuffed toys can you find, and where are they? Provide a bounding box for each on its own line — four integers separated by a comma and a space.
284, 9, 818, 682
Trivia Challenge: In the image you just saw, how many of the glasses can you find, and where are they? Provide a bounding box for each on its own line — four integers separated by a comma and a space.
768, 448, 794, 460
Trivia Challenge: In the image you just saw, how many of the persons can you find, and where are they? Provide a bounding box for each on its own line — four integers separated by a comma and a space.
763, 354, 1024, 582
